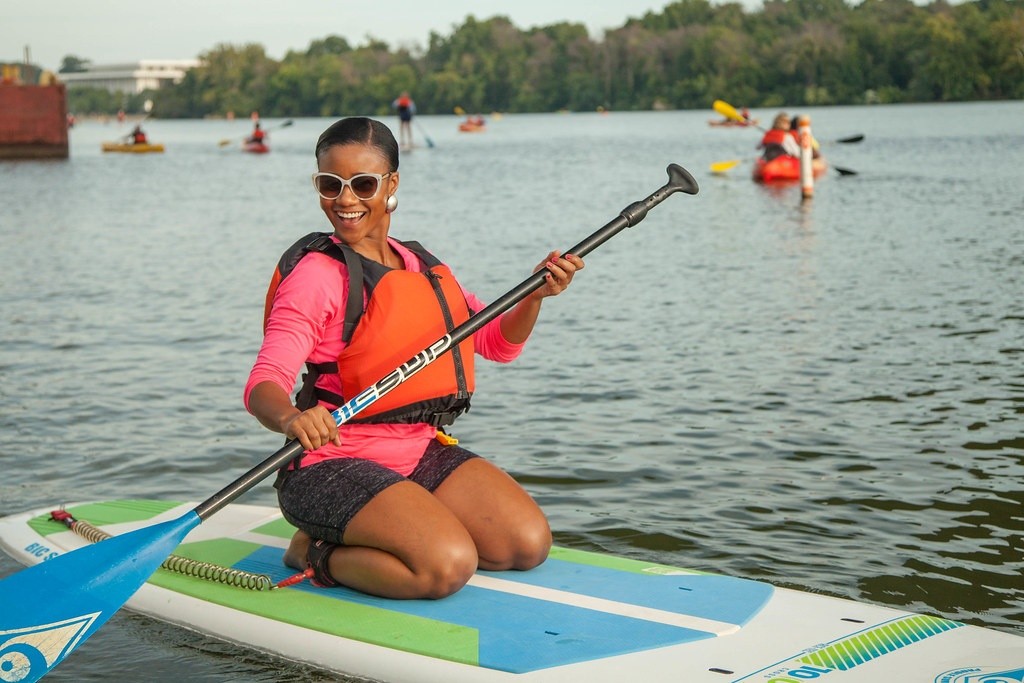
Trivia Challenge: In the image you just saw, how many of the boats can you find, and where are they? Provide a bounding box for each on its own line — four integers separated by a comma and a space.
459, 122, 486, 132
750, 154, 826, 184
101, 143, 165, 153
708, 120, 757, 127
241, 140, 267, 152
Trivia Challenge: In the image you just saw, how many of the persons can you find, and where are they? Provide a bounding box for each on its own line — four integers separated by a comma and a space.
130, 125, 146, 145
250, 123, 266, 143
742, 107, 749, 120
244, 118, 583, 601
756, 113, 821, 162
393, 90, 416, 146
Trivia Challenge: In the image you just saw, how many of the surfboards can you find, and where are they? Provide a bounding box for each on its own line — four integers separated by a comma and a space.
0, 491, 1024, 683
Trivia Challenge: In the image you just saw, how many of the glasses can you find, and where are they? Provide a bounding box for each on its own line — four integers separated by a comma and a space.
311, 172, 390, 201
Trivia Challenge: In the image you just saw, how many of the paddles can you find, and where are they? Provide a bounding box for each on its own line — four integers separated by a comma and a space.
411, 117, 435, 148
710, 133, 865, 175
219, 115, 293, 146
486, 112, 502, 122
454, 106, 467, 119
0, 160, 699, 683
712, 99, 858, 176
